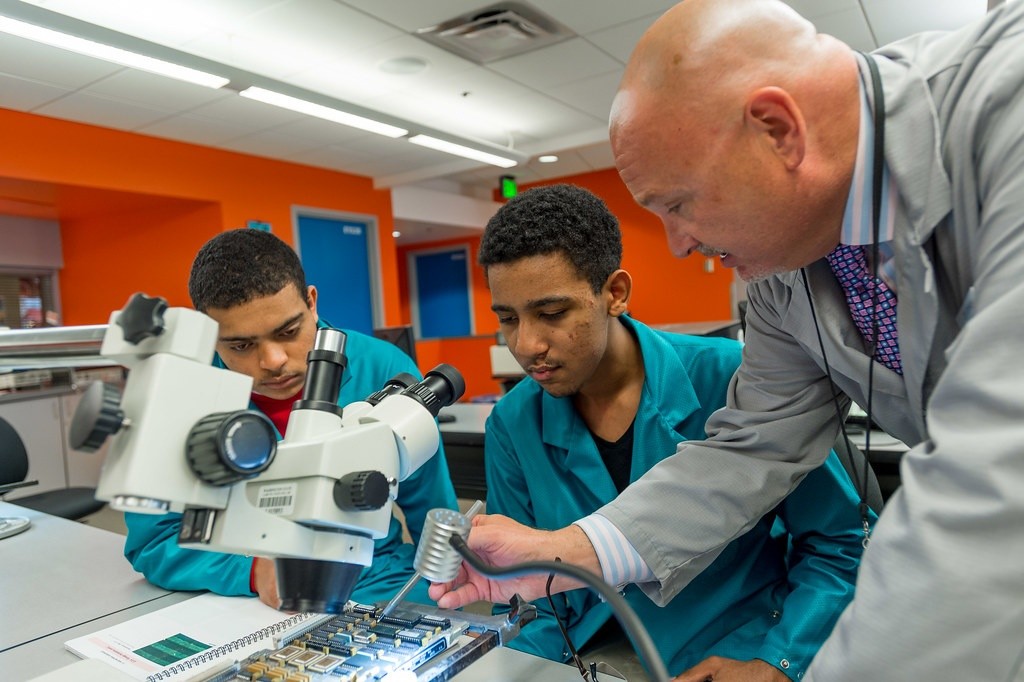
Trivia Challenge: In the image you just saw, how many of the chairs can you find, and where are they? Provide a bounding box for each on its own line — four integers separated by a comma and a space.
0, 416, 109, 520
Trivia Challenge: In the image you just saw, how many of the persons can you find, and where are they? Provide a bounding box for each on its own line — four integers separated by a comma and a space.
124, 229, 464, 612
429, 0, 1024, 682
486, 184, 879, 682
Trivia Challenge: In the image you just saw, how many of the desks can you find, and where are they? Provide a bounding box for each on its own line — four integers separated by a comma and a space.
0, 500, 623, 682
435, 400, 909, 466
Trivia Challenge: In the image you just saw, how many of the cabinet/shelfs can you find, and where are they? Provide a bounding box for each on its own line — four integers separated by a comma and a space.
0, 394, 112, 498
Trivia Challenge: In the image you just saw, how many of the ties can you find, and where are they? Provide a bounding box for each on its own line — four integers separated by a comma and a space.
825, 245, 906, 375
